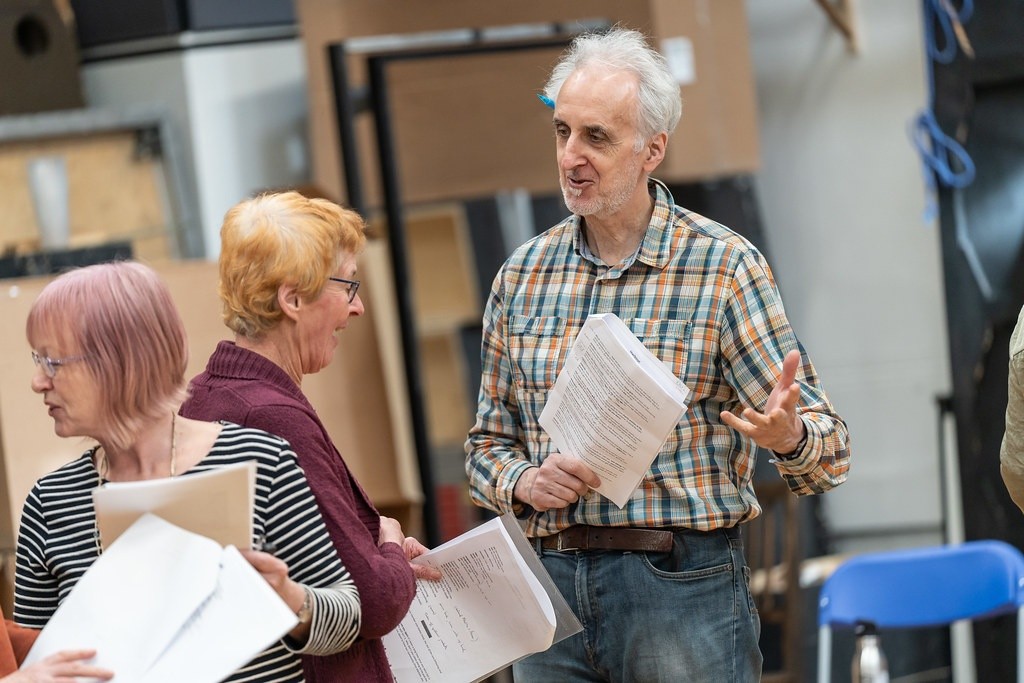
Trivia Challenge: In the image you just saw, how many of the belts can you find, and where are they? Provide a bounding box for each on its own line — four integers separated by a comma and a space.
527, 526, 673, 551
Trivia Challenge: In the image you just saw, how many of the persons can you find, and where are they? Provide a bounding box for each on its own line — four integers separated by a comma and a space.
176, 187, 444, 683
999, 304, 1024, 516
462, 18, 853, 683
0, 604, 115, 683
11, 253, 365, 683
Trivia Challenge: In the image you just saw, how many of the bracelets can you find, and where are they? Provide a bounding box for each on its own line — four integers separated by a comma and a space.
292, 587, 312, 633
774, 437, 809, 461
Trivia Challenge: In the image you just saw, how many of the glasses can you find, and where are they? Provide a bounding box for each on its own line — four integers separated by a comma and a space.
329, 277, 360, 303
32, 351, 89, 377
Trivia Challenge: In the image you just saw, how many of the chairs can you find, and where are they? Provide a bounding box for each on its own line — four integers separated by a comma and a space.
742, 478, 800, 682
818, 539, 1023, 682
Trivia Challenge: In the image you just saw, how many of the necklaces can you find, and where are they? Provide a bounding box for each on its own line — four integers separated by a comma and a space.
91, 409, 176, 558
